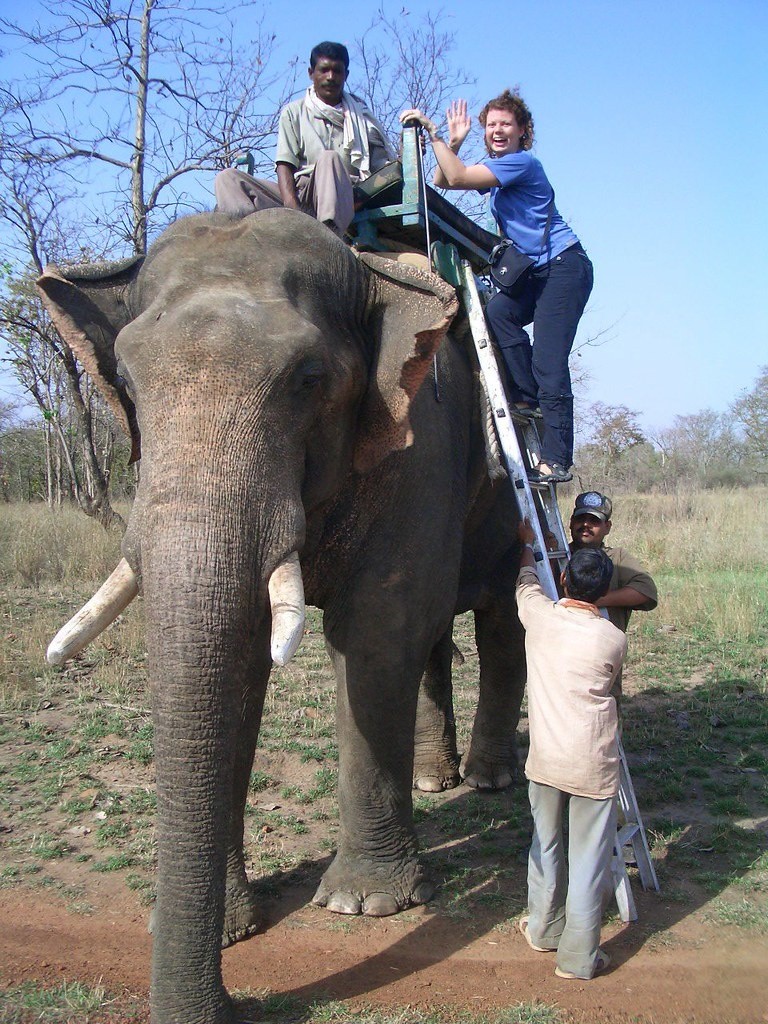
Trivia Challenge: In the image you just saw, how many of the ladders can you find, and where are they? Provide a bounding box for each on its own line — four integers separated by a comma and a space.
458, 256, 662, 923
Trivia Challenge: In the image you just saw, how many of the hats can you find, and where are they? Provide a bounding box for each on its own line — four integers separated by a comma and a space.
574, 491, 612, 520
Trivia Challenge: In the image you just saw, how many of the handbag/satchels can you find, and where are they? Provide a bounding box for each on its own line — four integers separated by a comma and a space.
487, 238, 538, 294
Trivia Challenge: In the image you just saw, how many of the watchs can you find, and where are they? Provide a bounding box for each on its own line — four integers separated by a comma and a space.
428, 128, 444, 142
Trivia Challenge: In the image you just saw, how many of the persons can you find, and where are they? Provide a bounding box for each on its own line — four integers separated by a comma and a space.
213, 40, 398, 241
399, 85, 594, 483
548, 491, 658, 740
512, 517, 630, 981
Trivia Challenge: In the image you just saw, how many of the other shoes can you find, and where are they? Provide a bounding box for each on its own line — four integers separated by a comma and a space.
518, 915, 551, 951
554, 947, 611, 978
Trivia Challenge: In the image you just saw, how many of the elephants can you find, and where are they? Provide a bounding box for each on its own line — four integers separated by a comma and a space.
35, 208, 561, 1024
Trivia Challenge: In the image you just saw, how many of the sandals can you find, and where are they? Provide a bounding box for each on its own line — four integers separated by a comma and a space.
508, 401, 543, 419
526, 458, 573, 483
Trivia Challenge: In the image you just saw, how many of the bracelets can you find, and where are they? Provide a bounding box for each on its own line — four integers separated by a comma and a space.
448, 144, 459, 155
523, 543, 534, 553
